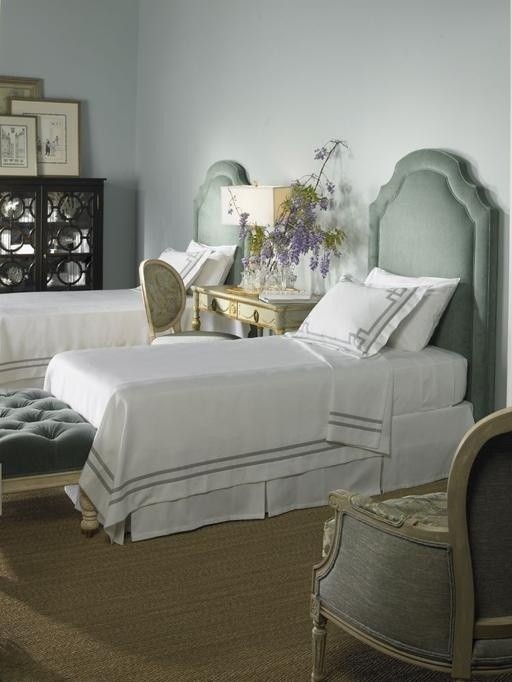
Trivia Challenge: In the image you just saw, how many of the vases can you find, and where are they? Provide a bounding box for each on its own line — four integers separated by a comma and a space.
262, 268, 292, 291
243, 268, 263, 291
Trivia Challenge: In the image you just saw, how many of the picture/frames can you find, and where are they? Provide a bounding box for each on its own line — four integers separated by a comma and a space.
0, 114, 39, 176
0, 75, 44, 115
8, 96, 83, 178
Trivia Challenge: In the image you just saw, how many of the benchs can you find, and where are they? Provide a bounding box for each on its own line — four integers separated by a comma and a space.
1, 390, 98, 539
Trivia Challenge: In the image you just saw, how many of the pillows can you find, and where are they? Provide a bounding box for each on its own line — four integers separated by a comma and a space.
138, 247, 214, 292
185, 240, 237, 286
292, 273, 434, 359
365, 266, 460, 354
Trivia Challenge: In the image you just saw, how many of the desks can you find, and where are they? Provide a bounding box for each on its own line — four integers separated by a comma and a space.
189, 284, 319, 336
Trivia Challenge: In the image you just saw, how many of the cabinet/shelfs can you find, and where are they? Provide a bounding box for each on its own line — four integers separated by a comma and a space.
0, 177, 107, 294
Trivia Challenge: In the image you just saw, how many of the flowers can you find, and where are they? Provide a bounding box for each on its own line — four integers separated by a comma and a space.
226, 139, 352, 278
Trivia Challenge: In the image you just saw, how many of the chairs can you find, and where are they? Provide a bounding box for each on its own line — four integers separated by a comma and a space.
140, 259, 239, 345
310, 405, 512, 682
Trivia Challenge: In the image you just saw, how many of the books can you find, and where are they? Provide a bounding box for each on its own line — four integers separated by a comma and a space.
258, 293, 318, 305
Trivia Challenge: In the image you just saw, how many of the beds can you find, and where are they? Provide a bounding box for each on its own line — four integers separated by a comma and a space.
0, 160, 253, 390
43, 149, 503, 545
